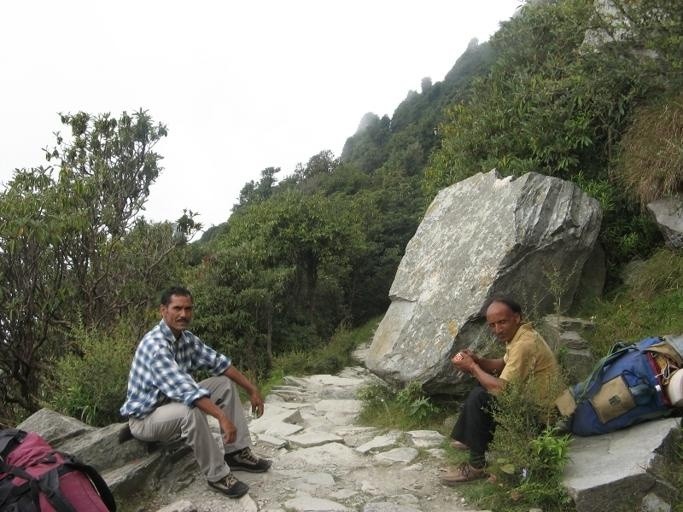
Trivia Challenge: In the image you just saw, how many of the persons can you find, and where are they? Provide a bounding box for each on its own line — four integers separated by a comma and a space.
114, 286, 273, 500
436, 296, 576, 490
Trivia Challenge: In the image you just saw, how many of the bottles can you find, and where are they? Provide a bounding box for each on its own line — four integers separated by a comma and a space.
628, 383, 661, 407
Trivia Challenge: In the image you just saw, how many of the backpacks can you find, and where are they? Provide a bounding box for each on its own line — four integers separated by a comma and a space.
0, 426, 115, 511
556, 334, 683, 436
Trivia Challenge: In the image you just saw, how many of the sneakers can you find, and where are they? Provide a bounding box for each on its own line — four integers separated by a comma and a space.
224, 447, 271, 472
452, 440, 471, 450
207, 472, 249, 498
441, 464, 489, 484
